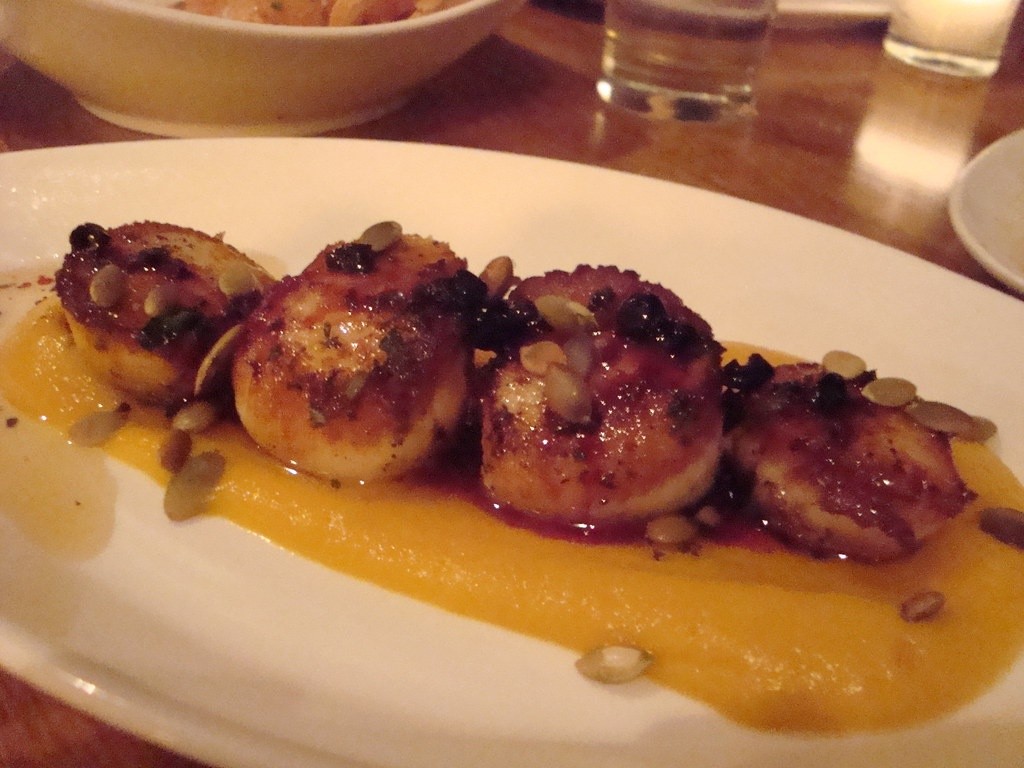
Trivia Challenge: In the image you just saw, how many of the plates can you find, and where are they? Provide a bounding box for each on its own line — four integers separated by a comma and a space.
0, 0, 529, 143
946, 120, 1024, 297
0, 125, 1024, 768
628, 0, 892, 37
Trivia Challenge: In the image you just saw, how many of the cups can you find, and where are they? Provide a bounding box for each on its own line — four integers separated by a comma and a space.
881, 0, 1023, 79
598, 0, 777, 122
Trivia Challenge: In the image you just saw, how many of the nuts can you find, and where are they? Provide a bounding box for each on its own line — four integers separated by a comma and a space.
69, 219, 1022, 682
